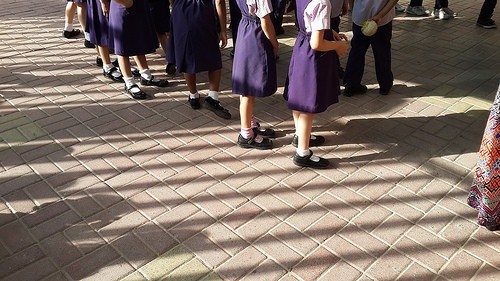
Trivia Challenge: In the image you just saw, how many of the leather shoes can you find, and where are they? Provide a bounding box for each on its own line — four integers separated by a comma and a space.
104, 67, 125, 83
238, 132, 274, 150
84, 39, 95, 48
252, 122, 276, 137
141, 75, 168, 87
292, 149, 330, 169
96, 56, 119, 67
64, 29, 80, 39
292, 135, 325, 148
125, 84, 147, 99
204, 96, 231, 119
131, 69, 141, 76
188, 94, 201, 110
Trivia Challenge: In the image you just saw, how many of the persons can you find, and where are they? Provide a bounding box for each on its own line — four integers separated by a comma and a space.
283, 0, 352, 168
108, 0, 168, 99
231, 0, 279, 149
63, 0, 176, 84
166, 0, 231, 119
229, 0, 349, 78
344, 0, 400, 98
395, 0, 498, 29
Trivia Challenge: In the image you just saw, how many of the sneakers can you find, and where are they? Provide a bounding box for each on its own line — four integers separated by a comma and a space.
431, 8, 450, 20
395, 3, 406, 12
379, 81, 393, 95
443, 8, 458, 17
476, 17, 497, 28
344, 84, 367, 97
405, 4, 430, 16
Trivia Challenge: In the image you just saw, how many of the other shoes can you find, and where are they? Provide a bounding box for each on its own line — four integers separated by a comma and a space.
166, 63, 177, 74
